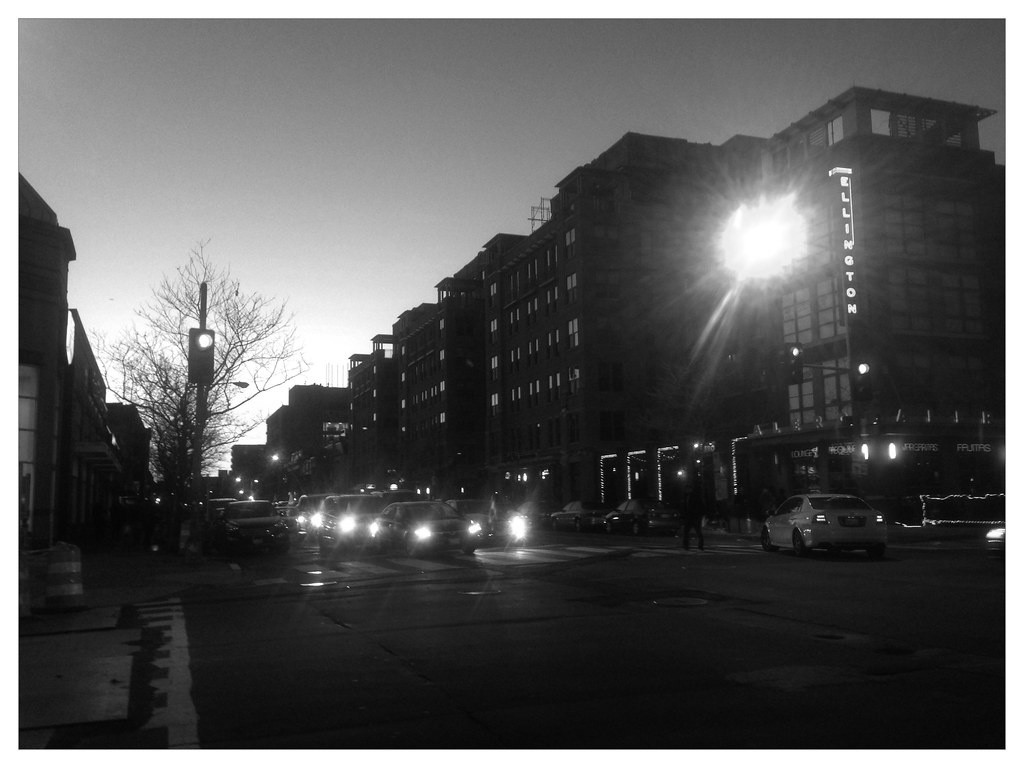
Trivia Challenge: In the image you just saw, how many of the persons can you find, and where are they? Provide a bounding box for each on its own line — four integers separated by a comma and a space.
678, 486, 707, 550
94, 498, 156, 544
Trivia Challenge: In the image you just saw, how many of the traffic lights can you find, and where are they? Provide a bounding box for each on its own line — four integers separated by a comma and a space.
854, 363, 872, 401
189, 328, 214, 383
784, 343, 803, 385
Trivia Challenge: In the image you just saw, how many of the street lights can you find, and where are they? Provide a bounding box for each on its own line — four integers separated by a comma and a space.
771, 226, 857, 401
181, 382, 249, 470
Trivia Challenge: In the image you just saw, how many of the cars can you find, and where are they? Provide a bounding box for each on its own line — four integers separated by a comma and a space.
983, 528, 1005, 576
273, 490, 419, 549
602, 497, 679, 537
209, 498, 237, 517
210, 500, 289, 556
445, 499, 529, 546
761, 494, 888, 557
365, 501, 483, 555
541, 501, 614, 532
